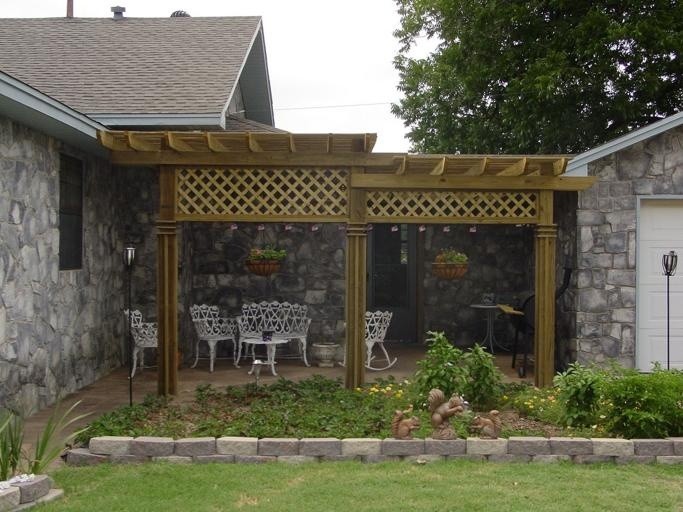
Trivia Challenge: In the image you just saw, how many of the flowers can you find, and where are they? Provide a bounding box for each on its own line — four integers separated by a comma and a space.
248, 240, 289, 264
434, 246, 468, 265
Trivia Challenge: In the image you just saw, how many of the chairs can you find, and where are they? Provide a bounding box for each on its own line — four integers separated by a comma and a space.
124, 309, 158, 379
338, 310, 397, 371
189, 304, 240, 373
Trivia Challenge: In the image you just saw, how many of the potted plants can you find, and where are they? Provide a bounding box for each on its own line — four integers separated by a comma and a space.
312, 336, 341, 368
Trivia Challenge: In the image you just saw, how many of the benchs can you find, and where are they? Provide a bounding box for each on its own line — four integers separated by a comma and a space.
233, 300, 312, 368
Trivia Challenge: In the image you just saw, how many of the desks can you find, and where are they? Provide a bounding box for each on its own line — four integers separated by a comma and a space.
244, 338, 288, 376
471, 302, 509, 355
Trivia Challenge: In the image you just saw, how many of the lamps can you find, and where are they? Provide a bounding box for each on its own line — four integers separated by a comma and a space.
123, 246, 137, 271
661, 251, 678, 276
